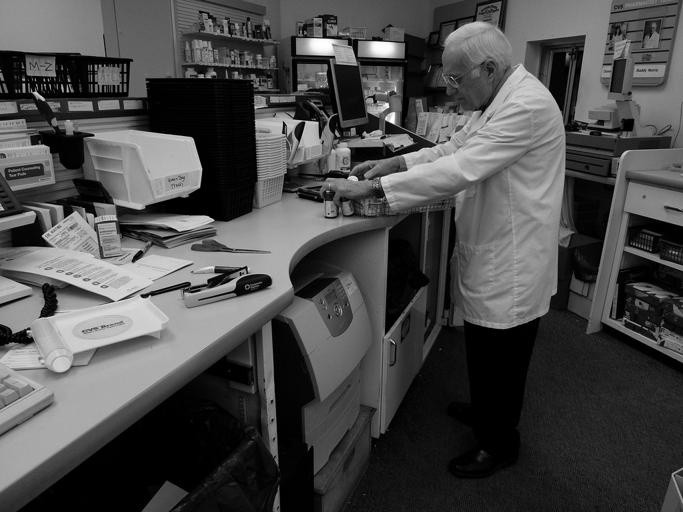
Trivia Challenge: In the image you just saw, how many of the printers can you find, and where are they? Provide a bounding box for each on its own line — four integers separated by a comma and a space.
269, 262, 374, 474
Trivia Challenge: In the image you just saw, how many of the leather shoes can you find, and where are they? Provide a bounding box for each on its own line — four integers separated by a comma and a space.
449, 430, 521, 480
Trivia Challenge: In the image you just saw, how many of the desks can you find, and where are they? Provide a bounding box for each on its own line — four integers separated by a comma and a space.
0, 188, 451, 509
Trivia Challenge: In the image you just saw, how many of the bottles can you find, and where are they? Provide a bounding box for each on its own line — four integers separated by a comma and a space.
323, 190, 356, 219
255, 54, 276, 70
266, 72, 273, 88
334, 141, 352, 171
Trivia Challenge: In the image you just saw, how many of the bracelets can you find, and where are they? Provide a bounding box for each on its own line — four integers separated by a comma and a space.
371, 176, 383, 198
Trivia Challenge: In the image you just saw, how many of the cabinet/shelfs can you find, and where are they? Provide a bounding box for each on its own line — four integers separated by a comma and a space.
565, 146, 682, 364
181, 32, 281, 94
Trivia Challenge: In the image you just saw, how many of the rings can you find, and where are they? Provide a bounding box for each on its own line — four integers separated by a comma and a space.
328, 183, 330, 191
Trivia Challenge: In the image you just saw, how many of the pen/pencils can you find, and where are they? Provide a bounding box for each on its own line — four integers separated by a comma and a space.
140, 282, 190, 298
193, 266, 239, 273
132, 241, 152, 263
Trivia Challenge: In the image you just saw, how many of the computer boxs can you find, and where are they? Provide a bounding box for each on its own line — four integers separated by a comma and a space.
338, 133, 419, 171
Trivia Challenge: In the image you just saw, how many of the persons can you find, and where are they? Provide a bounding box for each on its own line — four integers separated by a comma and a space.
317, 22, 566, 477
642, 22, 659, 49
610, 25, 624, 44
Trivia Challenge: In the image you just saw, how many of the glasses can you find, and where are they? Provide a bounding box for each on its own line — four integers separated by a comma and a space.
442, 67, 476, 88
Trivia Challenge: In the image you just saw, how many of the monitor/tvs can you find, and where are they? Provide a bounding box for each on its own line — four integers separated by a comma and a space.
325, 57, 370, 140
607, 57, 635, 102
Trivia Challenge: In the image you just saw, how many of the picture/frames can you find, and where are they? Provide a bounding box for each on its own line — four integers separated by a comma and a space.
428, 0, 506, 49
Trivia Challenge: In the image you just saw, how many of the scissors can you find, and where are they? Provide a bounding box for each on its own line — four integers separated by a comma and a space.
191, 239, 272, 254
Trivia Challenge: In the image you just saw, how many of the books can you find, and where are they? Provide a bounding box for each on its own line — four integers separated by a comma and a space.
0, 309, 95, 371
116, 213, 217, 249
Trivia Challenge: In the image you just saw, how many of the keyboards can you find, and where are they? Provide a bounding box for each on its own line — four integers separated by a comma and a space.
0, 360, 56, 438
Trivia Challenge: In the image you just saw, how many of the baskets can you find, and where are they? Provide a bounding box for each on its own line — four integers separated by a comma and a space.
252, 132, 288, 209
626, 226, 683, 266
355, 196, 453, 216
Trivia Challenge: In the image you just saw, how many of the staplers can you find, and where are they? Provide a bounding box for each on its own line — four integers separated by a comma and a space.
181, 266, 272, 308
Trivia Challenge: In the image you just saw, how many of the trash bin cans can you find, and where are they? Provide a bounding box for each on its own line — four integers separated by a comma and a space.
21, 393, 261, 512
